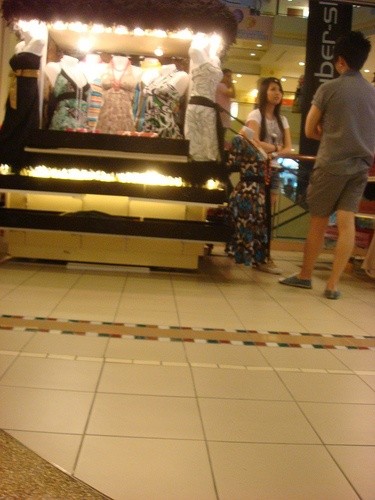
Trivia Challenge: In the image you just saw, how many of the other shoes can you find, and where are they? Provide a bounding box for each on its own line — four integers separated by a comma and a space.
278, 273, 312, 289
323, 286, 342, 299
253, 260, 284, 275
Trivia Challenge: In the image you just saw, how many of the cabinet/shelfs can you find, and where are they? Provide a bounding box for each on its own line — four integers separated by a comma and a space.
0, 130, 229, 270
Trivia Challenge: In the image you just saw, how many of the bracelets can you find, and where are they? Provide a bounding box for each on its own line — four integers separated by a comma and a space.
273, 144, 278, 151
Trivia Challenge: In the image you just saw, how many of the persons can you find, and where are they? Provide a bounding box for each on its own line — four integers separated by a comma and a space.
184, 36, 223, 164
215, 68, 236, 138
0, 18, 45, 176
44, 52, 189, 139
243, 77, 293, 274
278, 30, 375, 300
217, 127, 271, 268
